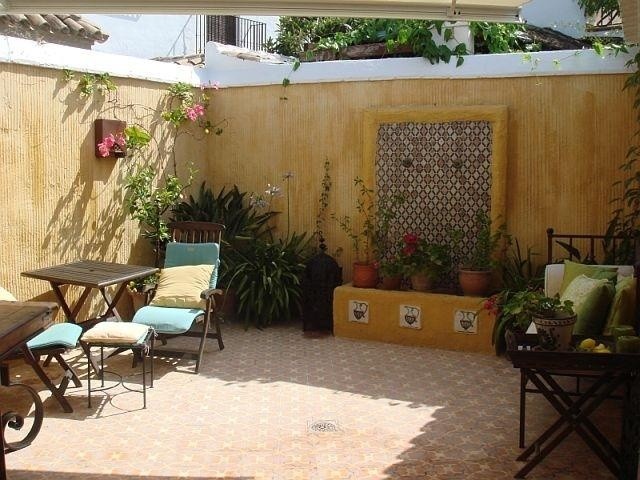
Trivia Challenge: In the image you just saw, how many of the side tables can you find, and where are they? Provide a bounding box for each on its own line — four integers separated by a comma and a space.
512, 352, 639, 478
520, 368, 637, 448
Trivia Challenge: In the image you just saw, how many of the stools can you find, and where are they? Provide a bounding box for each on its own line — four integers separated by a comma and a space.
87, 329, 155, 409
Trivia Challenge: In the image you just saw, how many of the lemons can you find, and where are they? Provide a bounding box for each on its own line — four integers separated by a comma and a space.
580, 338, 596, 350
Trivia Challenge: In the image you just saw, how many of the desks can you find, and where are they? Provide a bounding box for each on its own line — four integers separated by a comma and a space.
21, 259, 160, 379
0, 302, 59, 366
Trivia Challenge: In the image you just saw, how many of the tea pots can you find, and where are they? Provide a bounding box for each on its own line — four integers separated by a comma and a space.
404, 306, 420, 325
352, 301, 368, 320
460, 311, 475, 330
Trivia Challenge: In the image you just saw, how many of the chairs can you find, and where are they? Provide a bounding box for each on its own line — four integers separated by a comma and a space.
132, 222, 226, 374
0, 323, 83, 413
543, 263, 635, 298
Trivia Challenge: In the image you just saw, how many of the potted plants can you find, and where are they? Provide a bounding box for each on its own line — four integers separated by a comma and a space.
330, 176, 405, 290
457, 207, 513, 294
170, 181, 282, 318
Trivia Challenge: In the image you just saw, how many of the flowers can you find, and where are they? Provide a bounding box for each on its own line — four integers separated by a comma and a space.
63, 69, 228, 296
391, 229, 462, 284
226, 170, 316, 332
471, 287, 575, 357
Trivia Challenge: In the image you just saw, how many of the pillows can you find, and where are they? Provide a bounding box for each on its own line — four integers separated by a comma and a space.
148, 264, 215, 312
558, 260, 637, 335
81, 322, 148, 344
0, 287, 18, 301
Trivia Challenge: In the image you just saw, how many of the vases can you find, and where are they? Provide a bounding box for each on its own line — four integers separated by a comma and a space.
133, 295, 151, 313
531, 312, 577, 351
409, 271, 432, 290
